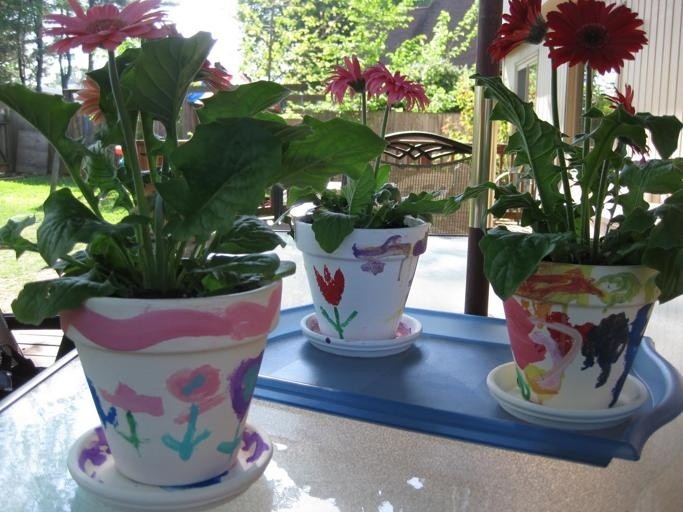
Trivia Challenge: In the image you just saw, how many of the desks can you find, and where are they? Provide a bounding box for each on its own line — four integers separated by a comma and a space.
0, 302, 680, 512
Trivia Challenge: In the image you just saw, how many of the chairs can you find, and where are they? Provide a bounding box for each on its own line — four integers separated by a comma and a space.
491, 141, 541, 224
343, 130, 474, 238
131, 136, 285, 222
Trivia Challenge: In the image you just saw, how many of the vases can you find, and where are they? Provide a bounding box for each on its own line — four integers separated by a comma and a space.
58, 237, 283, 507
485, 248, 650, 428
292, 196, 430, 358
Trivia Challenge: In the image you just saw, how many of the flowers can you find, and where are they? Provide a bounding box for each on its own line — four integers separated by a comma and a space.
457, 0, 680, 302
274, 57, 461, 255
0, 1, 384, 324
